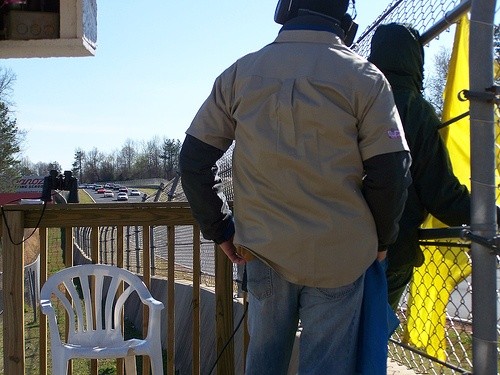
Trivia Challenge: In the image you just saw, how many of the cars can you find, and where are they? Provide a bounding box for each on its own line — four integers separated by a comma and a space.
78, 182, 141, 201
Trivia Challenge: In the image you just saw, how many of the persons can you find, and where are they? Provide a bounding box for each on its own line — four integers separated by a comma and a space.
179, 0, 413, 375
356, 22, 500, 375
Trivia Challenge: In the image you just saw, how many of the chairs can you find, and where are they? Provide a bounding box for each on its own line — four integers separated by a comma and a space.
39, 264, 165, 375
